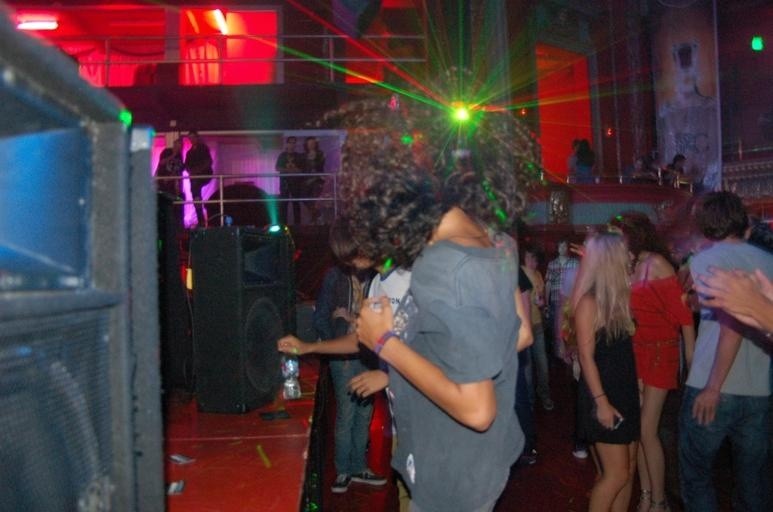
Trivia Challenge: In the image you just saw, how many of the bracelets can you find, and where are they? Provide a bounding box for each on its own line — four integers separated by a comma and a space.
373, 331, 397, 356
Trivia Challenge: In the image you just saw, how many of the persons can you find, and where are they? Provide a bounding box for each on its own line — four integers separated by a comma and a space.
155, 137, 184, 228
277, 136, 325, 227
182, 128, 216, 227
622, 153, 705, 194
317, 253, 388, 494
614, 211, 694, 508
276, 71, 544, 510
522, 240, 589, 459
568, 138, 599, 185
690, 266, 770, 334
559, 234, 640, 508
276, 212, 535, 508
676, 194, 770, 509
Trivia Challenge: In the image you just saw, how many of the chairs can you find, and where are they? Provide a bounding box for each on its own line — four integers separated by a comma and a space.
350, 469, 387, 486
573, 442, 589, 459
330, 472, 350, 493
543, 397, 553, 410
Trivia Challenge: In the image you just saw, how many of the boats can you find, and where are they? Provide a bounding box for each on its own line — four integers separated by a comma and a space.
0, 12, 164, 512
190, 227, 296, 413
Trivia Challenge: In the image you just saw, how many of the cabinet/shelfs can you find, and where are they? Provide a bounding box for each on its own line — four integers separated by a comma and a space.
280, 352, 302, 400
